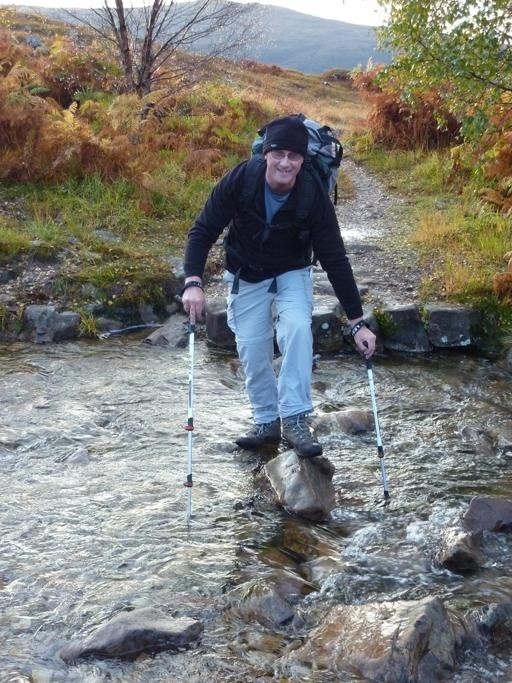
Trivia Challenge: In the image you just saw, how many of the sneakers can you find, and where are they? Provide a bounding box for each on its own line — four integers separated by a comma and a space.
236, 417, 281, 449
281, 411, 322, 457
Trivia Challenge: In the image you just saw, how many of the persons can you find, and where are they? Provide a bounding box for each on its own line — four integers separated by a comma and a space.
180, 114, 377, 459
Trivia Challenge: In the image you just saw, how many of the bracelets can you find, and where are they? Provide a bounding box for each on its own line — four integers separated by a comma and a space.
349, 318, 370, 337
180, 280, 205, 295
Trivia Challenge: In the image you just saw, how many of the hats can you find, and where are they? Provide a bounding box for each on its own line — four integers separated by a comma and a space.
262, 115, 308, 160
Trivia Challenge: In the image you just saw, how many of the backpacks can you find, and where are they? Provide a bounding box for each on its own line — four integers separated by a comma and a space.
250, 112, 343, 204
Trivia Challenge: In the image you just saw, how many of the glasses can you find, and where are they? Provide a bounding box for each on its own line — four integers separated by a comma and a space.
271, 149, 300, 160
